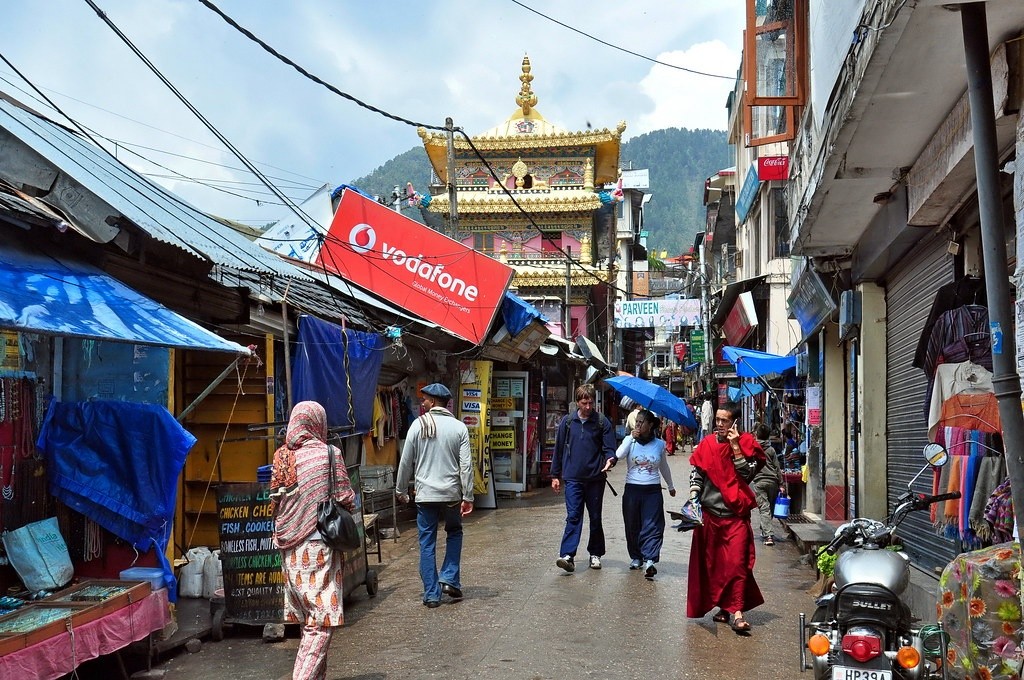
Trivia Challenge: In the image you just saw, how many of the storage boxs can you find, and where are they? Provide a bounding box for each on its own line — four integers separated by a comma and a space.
0, 567, 166, 656
257, 465, 272, 481
359, 465, 394, 490
542, 451, 563, 485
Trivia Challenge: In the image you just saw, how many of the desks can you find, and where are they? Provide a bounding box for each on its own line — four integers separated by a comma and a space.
783, 473, 802, 484
0, 587, 172, 680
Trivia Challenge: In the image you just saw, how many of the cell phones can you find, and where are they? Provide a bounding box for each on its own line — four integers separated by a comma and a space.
730, 419, 740, 429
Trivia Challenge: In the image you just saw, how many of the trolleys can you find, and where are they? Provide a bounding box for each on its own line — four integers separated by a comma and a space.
210, 419, 380, 642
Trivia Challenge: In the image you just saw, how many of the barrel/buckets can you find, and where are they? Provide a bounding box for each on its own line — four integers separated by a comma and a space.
203, 550, 224, 598
774, 492, 791, 519
179, 545, 211, 598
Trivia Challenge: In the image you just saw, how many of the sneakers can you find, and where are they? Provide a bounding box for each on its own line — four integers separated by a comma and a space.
555, 554, 575, 573
589, 555, 601, 568
643, 561, 658, 579
629, 559, 644, 569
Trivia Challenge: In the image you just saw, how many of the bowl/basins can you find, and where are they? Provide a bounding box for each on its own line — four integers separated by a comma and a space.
209, 597, 225, 616
214, 588, 224, 597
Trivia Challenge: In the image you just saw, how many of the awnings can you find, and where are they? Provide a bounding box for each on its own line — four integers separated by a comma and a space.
684, 361, 701, 372
0, 257, 254, 355
722, 346, 797, 403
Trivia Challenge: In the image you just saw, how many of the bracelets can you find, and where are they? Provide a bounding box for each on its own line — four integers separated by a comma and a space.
733, 453, 742, 455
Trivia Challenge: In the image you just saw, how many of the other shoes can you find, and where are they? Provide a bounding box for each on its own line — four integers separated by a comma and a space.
763, 537, 774, 546
682, 449, 685, 452
668, 453, 675, 456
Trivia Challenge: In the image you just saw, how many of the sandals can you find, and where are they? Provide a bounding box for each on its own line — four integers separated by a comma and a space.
712, 610, 730, 622
731, 618, 751, 630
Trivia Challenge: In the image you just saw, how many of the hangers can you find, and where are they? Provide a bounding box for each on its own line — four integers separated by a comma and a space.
941, 263, 1002, 458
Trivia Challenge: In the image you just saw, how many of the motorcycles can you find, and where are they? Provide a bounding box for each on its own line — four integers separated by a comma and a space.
797, 442, 961, 680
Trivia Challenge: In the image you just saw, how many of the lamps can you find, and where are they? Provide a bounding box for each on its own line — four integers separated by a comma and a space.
873, 192, 892, 205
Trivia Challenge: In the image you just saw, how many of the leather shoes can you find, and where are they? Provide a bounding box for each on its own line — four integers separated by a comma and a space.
442, 583, 462, 598
424, 599, 441, 608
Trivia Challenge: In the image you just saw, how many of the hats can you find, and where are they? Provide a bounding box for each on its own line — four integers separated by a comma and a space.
420, 382, 452, 398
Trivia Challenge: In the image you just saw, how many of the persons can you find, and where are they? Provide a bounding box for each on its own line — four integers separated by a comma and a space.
550, 384, 618, 572
688, 402, 766, 631
749, 425, 802, 545
615, 410, 675, 581
270, 399, 356, 680
395, 383, 474, 608
625, 392, 714, 456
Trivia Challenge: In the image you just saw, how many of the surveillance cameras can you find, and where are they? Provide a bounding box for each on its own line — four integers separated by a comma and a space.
643, 331, 654, 340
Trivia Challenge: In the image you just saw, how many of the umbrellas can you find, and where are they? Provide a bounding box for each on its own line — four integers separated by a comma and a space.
667, 494, 704, 533
602, 375, 697, 430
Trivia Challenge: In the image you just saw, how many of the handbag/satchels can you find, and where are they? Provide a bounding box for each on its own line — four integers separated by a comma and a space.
2, 516, 74, 592
316, 497, 361, 552
